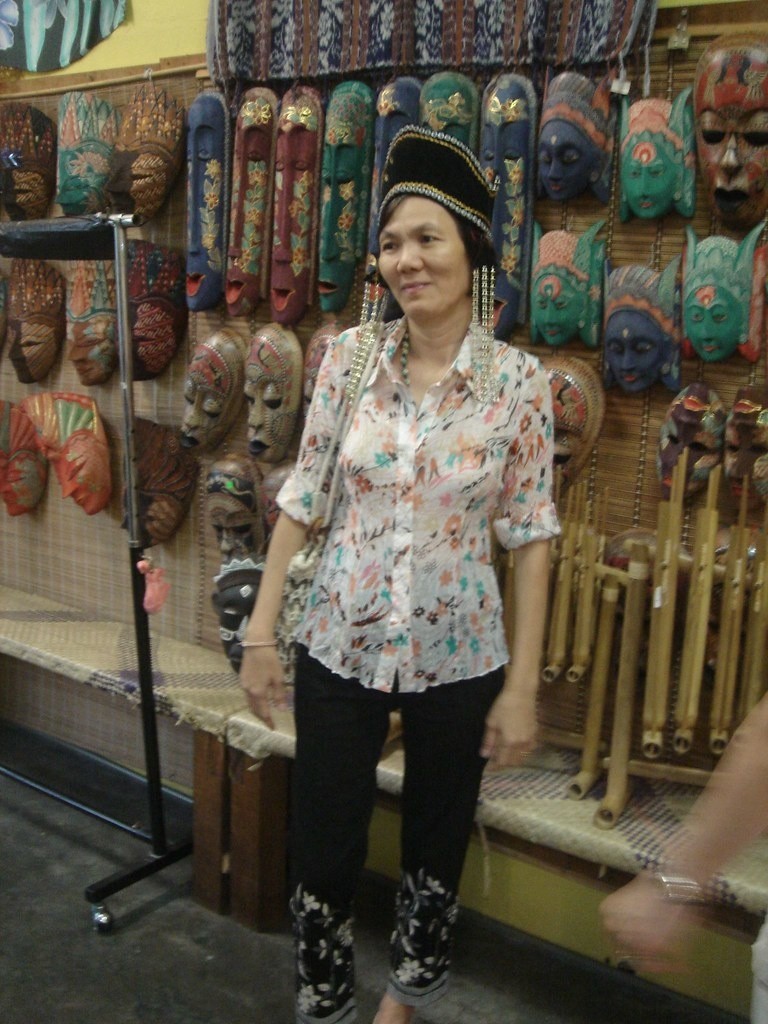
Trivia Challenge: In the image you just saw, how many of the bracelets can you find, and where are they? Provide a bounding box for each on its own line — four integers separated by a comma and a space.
241, 639, 279, 647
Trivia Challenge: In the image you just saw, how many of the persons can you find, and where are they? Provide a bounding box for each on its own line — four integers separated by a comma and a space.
597, 691, 768, 1024
239, 125, 561, 1024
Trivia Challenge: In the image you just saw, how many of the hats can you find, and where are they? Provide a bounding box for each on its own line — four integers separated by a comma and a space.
374, 123, 498, 241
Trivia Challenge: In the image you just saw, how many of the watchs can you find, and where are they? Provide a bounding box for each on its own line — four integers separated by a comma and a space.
647, 866, 706, 905
